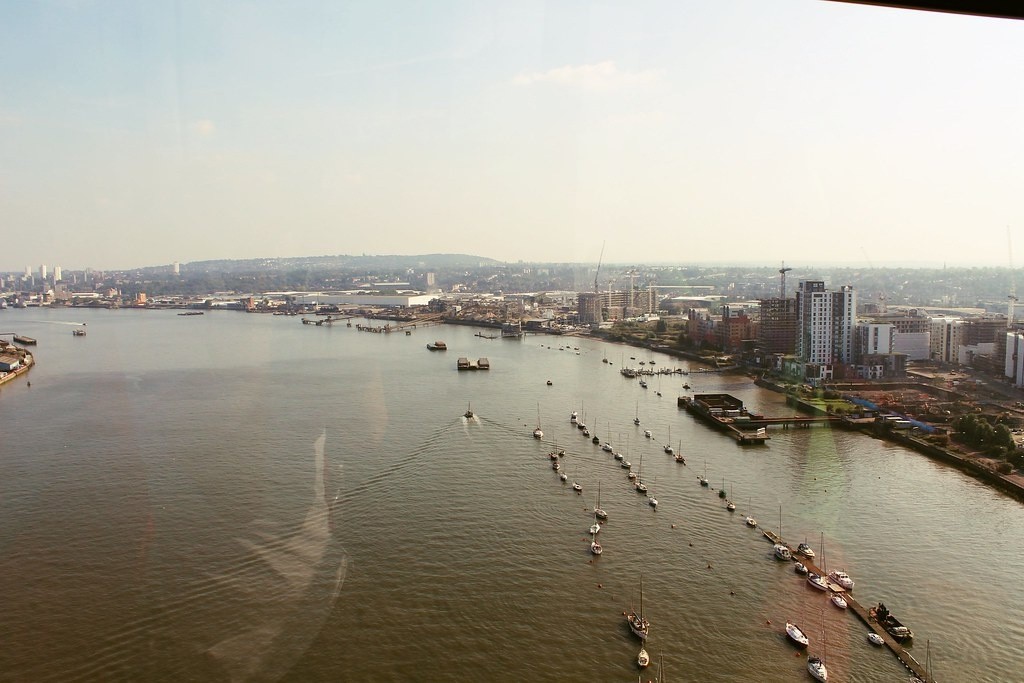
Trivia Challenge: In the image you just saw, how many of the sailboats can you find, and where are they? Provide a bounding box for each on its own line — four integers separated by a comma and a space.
649, 475, 659, 507
615, 433, 624, 459
719, 477, 726, 498
807, 532, 828, 592
464, 401, 473, 419
635, 453, 647, 493
633, 399, 640, 425
807, 605, 828, 682
746, 495, 757, 526
533, 401, 543, 438
602, 421, 613, 452
594, 481, 608, 520
583, 412, 589, 436
578, 400, 585, 429
727, 483, 736, 511
590, 509, 602, 555
628, 573, 650, 640
550, 429, 566, 462
663, 425, 674, 453
676, 439, 685, 463
603, 348, 607, 363
773, 504, 791, 561
592, 417, 599, 444
621, 433, 631, 468
701, 460, 709, 486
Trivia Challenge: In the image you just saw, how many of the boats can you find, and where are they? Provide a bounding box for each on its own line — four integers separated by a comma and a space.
868, 607, 915, 644
574, 347, 579, 350
828, 569, 855, 590
630, 357, 635, 360
867, 632, 884, 646
546, 381, 552, 385
640, 379, 647, 389
639, 361, 645, 365
797, 537, 815, 558
785, 620, 809, 650
620, 366, 688, 378
560, 473, 568, 481
576, 352, 580, 355
589, 521, 600, 534
795, 561, 809, 574
566, 346, 570, 348
571, 482, 583, 491
73, 329, 86, 336
644, 430, 652, 437
559, 347, 563, 350
83, 323, 87, 326
650, 361, 655, 364
830, 594, 847, 610
552, 462, 560, 469
570, 411, 578, 423
637, 648, 650, 668
628, 470, 636, 478
178, 311, 203, 316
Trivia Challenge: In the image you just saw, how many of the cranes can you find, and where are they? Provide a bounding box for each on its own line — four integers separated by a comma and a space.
779, 262, 792, 298
